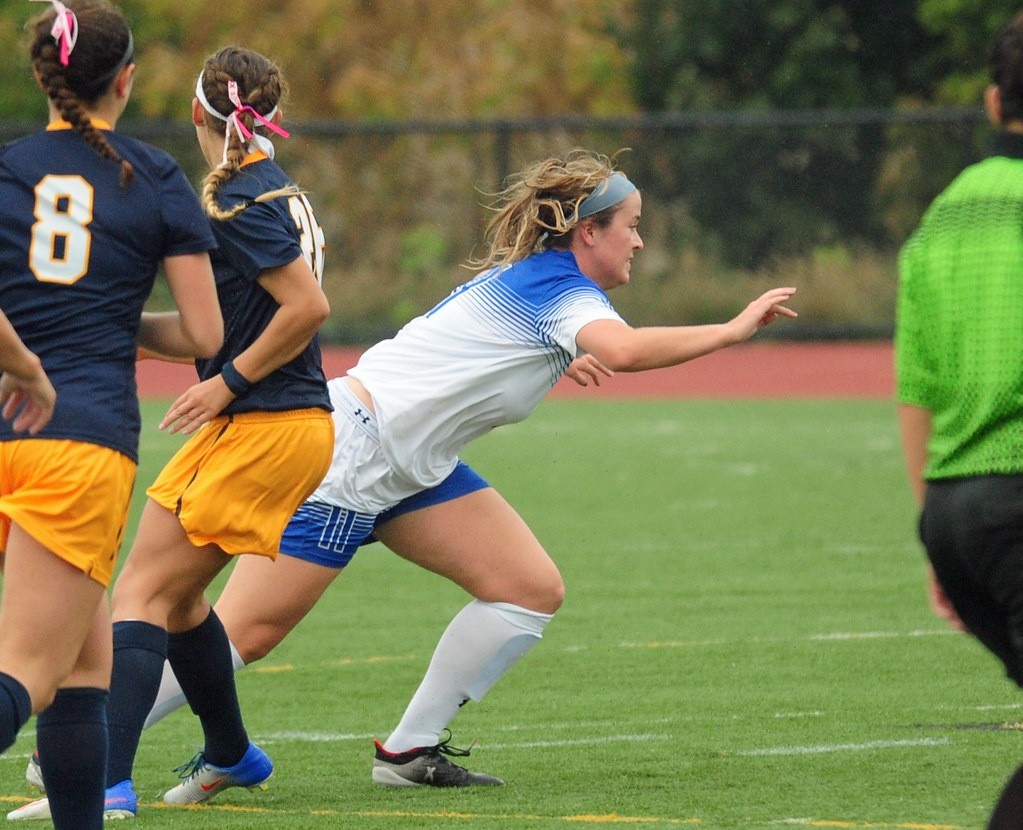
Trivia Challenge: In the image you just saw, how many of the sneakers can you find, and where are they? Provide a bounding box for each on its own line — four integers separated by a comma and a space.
104, 779, 139, 820
163, 742, 273, 805
26, 751, 43, 787
371, 728, 505, 789
6, 797, 51, 821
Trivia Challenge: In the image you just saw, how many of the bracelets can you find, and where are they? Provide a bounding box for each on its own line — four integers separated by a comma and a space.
220, 361, 253, 396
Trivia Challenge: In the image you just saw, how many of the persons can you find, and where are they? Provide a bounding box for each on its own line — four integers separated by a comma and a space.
0, 44, 336, 823
1, 0, 226, 830
893, 10, 1023, 830
23, 150, 801, 780
